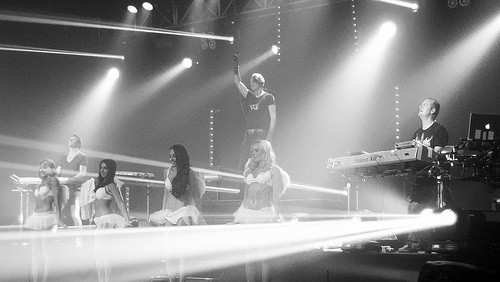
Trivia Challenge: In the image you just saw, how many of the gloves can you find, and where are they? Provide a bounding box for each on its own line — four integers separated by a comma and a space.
233, 52, 240, 75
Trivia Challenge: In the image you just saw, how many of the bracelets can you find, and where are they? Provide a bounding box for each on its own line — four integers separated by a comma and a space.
268, 130, 274, 134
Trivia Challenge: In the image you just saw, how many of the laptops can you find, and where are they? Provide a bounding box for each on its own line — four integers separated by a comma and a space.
468, 113, 500, 141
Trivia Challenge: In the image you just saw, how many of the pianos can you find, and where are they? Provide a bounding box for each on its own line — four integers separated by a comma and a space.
327, 139, 445, 177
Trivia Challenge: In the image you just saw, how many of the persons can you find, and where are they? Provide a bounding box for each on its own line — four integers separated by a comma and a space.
233, 139, 284, 282
24, 159, 66, 282
397, 98, 449, 253
149, 144, 201, 282
232, 51, 277, 175
55, 135, 89, 226
92, 159, 138, 282
